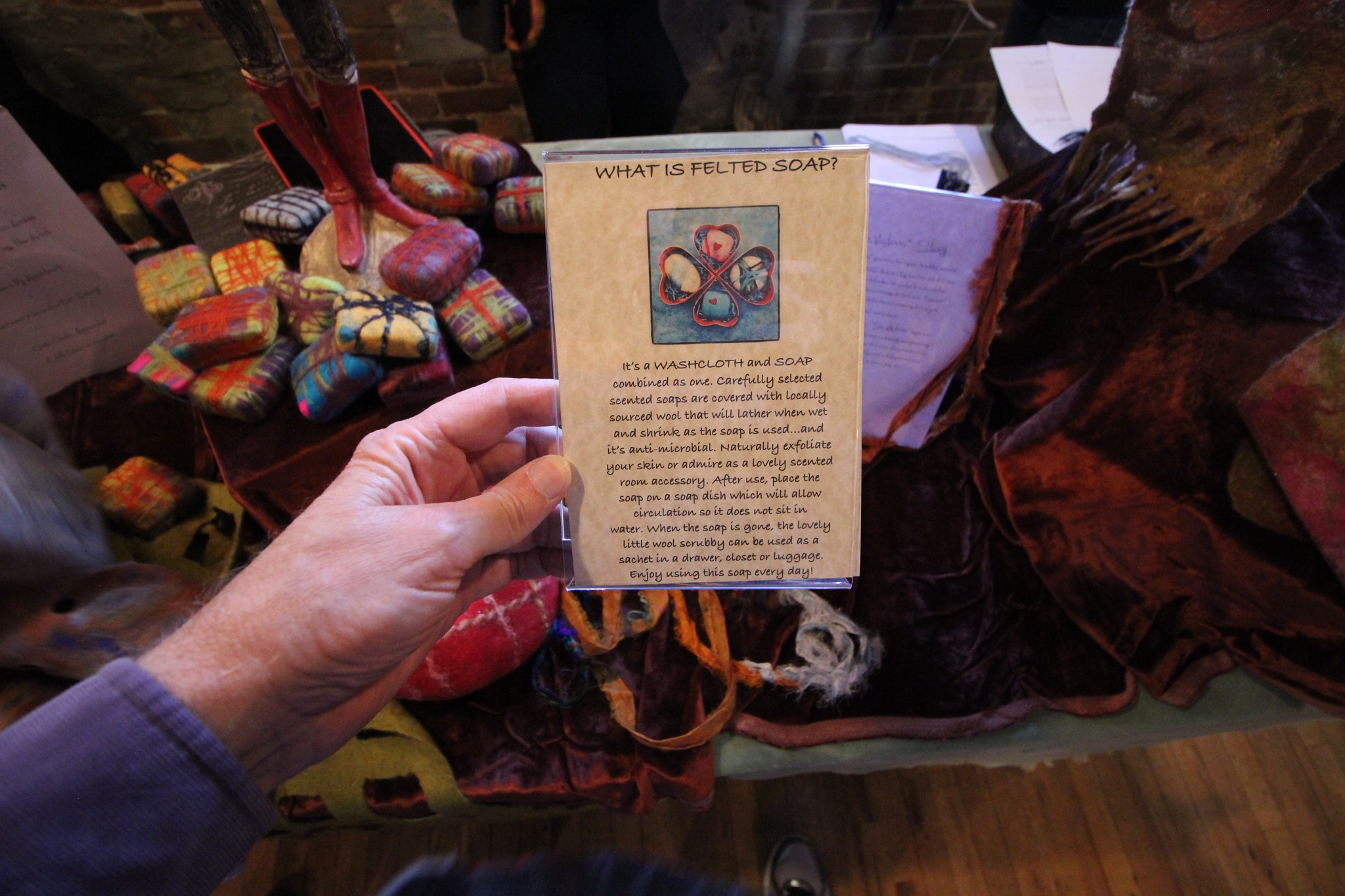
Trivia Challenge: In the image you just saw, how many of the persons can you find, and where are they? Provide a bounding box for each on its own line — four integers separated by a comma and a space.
200, 1, 440, 269
0, 376, 830, 896
